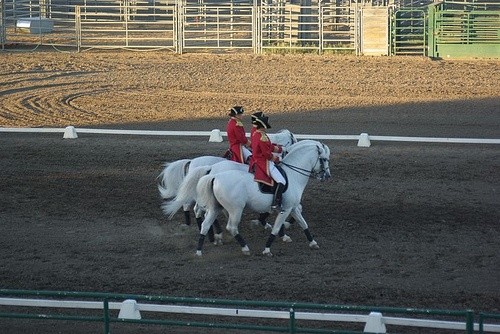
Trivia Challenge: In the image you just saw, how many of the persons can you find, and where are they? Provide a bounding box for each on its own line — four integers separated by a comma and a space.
223, 106, 251, 164
249, 111, 265, 148
249, 116, 287, 212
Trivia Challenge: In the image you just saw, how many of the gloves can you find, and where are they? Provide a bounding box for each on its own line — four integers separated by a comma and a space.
277, 156, 282, 163
281, 146, 285, 152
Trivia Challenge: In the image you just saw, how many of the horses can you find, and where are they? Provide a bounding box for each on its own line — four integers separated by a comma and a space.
155, 127, 333, 259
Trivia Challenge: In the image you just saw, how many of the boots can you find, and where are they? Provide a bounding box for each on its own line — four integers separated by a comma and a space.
271, 183, 285, 210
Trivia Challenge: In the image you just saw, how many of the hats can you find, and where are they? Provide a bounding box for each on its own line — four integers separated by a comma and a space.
252, 112, 262, 124
228, 106, 244, 116
256, 117, 271, 130
